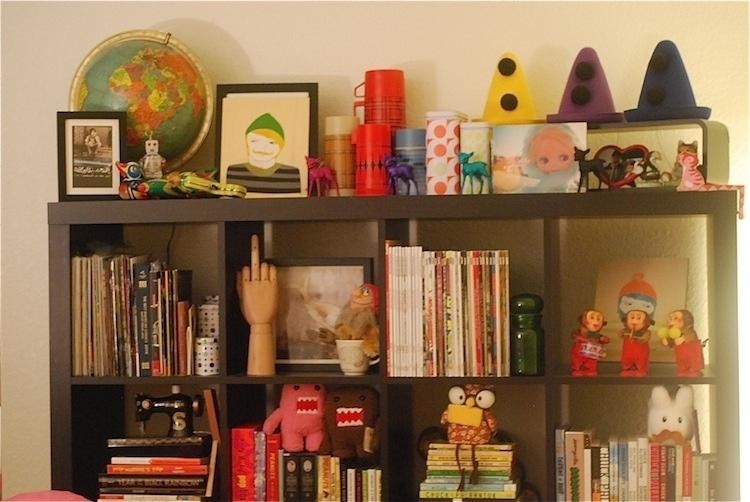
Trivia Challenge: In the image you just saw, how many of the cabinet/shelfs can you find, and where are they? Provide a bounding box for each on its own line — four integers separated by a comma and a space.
41, 183, 741, 500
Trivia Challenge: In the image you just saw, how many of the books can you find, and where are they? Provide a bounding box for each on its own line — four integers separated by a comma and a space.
230, 424, 382, 502
418, 439, 521, 501
383, 237, 512, 379
70, 253, 220, 380
555, 422, 717, 502
96, 456, 208, 502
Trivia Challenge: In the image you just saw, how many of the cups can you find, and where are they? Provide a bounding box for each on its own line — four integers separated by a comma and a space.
195, 297, 219, 376
335, 338, 381, 377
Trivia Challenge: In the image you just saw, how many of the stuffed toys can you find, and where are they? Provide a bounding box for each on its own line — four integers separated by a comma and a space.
263, 383, 325, 454
569, 306, 709, 377
335, 282, 381, 358
647, 386, 695, 444
323, 385, 380, 465
440, 383, 498, 492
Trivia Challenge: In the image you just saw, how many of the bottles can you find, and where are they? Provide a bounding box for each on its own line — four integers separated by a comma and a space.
511, 294, 544, 376
324, 71, 426, 197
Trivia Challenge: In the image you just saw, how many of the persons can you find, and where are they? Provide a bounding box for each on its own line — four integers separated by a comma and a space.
236, 234, 280, 377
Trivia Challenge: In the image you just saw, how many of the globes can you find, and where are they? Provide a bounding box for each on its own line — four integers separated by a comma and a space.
68, 29, 214, 176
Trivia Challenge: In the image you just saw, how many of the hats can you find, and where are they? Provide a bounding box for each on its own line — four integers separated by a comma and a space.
546, 47, 625, 123
470, 49, 545, 126
625, 40, 711, 122
245, 112, 285, 138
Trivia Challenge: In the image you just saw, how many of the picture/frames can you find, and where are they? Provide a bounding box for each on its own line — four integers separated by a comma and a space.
210, 80, 320, 199
54, 106, 128, 203
260, 254, 375, 374
